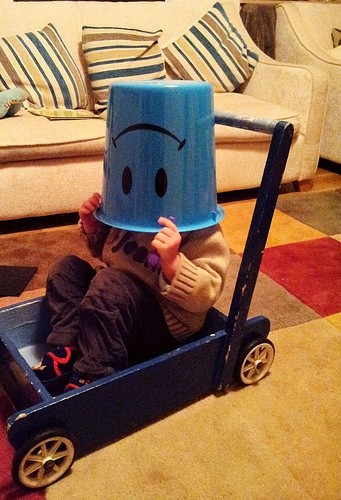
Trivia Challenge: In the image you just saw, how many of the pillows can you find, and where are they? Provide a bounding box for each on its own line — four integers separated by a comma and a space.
0, 23, 87, 110
161, 2, 258, 92
81, 26, 166, 111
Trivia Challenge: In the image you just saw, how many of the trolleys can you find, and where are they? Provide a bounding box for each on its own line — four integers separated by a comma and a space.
0, 104, 295, 492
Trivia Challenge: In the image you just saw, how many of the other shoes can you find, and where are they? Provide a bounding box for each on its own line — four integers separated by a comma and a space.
30, 344, 97, 394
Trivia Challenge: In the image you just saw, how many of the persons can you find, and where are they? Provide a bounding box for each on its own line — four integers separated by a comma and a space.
30, 80, 230, 394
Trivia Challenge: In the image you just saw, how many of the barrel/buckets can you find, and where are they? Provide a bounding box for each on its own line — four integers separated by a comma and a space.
93, 81, 225, 234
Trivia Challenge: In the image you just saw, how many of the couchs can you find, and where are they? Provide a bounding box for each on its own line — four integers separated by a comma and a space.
0, 0, 341, 222
